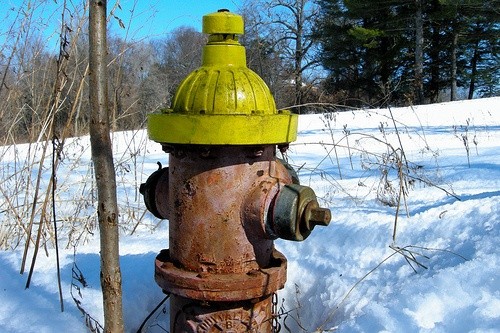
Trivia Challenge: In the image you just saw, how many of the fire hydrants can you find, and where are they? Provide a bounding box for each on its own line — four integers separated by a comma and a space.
139, 10, 330, 333
